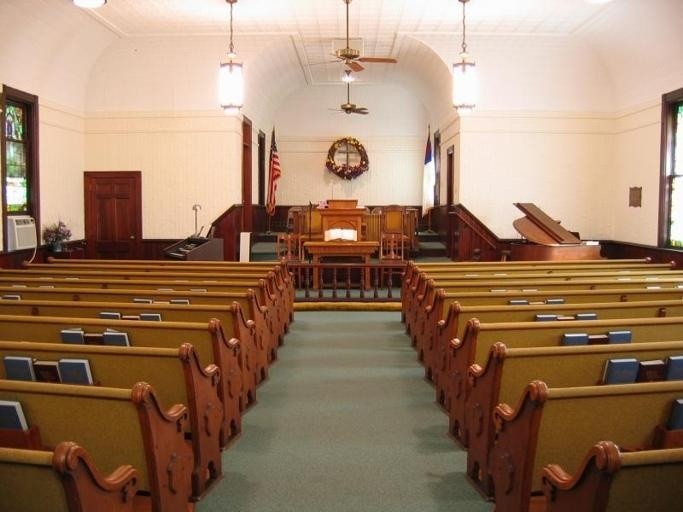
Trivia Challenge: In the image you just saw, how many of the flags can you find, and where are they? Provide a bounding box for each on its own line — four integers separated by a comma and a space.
266, 129, 281, 217
422, 130, 435, 219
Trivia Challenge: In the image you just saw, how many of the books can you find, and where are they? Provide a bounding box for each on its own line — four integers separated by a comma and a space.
0, 277, 216, 431
466, 273, 683, 432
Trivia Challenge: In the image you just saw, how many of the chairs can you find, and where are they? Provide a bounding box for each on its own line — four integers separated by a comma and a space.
379, 232, 408, 290
277, 232, 302, 288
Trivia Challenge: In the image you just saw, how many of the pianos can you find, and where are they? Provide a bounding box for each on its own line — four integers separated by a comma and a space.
510, 203, 609, 262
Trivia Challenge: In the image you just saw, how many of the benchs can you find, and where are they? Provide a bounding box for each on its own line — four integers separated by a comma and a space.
0, 256, 298, 512
398, 258, 682, 512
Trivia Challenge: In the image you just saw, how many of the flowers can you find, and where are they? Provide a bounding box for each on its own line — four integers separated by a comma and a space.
43, 218, 73, 244
325, 135, 370, 181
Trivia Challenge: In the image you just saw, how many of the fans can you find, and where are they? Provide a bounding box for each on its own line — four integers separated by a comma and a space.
304, 0, 398, 115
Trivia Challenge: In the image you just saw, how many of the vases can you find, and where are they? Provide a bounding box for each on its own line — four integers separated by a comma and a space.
48, 242, 64, 253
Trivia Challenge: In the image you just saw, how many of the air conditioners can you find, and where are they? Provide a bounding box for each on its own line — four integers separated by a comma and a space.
8, 214, 38, 253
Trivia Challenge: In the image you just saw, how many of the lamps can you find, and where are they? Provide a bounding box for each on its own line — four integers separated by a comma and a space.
192, 203, 202, 237
217, 0, 245, 116
451, 0, 478, 116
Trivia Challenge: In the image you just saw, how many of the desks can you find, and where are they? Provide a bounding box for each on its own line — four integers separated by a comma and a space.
303, 241, 379, 290
